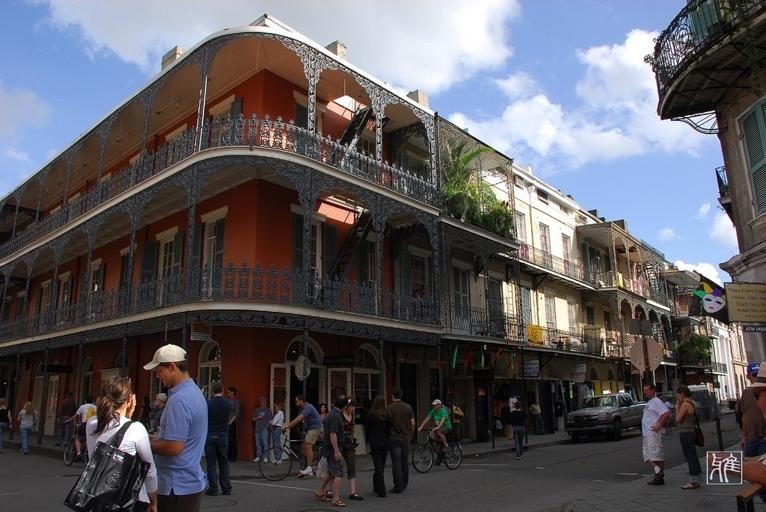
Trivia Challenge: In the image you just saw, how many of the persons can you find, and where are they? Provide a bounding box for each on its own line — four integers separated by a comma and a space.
449, 402, 464, 446
511, 402, 528, 459
529, 400, 545, 435
418, 399, 452, 466
641, 362, 766, 503
501, 401, 513, 439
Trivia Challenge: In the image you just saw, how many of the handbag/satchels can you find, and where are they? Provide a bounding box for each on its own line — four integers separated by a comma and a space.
63, 419, 151, 512
681, 400, 705, 448
32, 408, 37, 427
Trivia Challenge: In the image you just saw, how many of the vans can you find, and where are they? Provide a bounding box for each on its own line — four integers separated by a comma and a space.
656, 383, 712, 422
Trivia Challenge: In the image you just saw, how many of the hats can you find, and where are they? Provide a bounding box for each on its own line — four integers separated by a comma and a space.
142, 343, 188, 371
431, 399, 442, 405
747, 362, 761, 375
745, 361, 766, 388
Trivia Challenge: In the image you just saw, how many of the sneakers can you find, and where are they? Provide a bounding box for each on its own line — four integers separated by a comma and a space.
252, 457, 261, 463
442, 443, 449, 453
680, 482, 701, 489
74, 454, 81, 462
299, 466, 313, 476
204, 489, 231, 496
274, 459, 282, 465
647, 474, 665, 485
263, 457, 268, 464
511, 453, 524, 460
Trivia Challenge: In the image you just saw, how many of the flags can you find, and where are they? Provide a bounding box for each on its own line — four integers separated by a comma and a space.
688, 277, 730, 325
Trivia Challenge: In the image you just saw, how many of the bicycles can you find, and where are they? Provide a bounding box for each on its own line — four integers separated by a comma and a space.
256, 427, 325, 483
411, 430, 463, 475
57, 415, 91, 467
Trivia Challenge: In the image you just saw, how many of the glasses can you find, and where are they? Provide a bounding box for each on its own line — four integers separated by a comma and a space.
753, 389, 766, 401
749, 373, 758, 378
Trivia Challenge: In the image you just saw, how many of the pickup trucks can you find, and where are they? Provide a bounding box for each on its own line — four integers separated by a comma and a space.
566, 390, 647, 443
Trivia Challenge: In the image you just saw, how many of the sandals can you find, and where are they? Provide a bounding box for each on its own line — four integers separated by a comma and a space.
348, 493, 364, 501
314, 488, 347, 507
